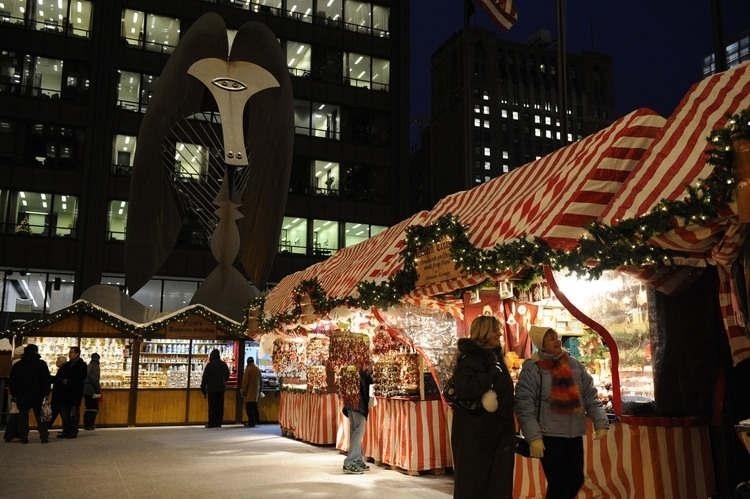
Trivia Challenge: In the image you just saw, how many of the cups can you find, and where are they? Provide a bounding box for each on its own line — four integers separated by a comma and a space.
40, 336, 131, 389
137, 371, 203, 388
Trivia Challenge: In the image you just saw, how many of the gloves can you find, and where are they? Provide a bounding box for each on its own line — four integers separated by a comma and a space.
482, 391, 498, 413
529, 439, 546, 458
592, 430, 608, 440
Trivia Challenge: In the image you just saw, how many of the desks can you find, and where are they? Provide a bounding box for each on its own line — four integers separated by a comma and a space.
512, 411, 715, 498
278, 384, 344, 446
363, 396, 455, 477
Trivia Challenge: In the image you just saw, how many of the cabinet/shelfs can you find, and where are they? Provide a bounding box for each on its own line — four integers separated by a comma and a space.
138, 341, 235, 364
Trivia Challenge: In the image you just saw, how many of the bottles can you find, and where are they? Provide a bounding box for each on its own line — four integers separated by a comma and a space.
137, 353, 205, 371
143, 343, 234, 356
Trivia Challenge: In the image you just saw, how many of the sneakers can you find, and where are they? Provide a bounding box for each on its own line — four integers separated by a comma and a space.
343, 462, 369, 474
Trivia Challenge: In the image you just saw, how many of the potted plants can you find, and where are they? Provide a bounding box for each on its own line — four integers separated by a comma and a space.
577, 326, 612, 388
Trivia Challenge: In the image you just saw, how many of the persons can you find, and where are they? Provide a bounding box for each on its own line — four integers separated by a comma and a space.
123, 344, 132, 373
200, 349, 230, 428
443, 316, 531, 498
343, 367, 374, 474
8, 342, 102, 445
239, 357, 263, 426
513, 326, 609, 498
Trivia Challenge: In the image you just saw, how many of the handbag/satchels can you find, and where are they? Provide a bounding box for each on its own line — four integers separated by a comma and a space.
443, 376, 482, 412
513, 434, 530, 457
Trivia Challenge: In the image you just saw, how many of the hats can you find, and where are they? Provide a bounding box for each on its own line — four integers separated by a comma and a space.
529, 326, 552, 351
